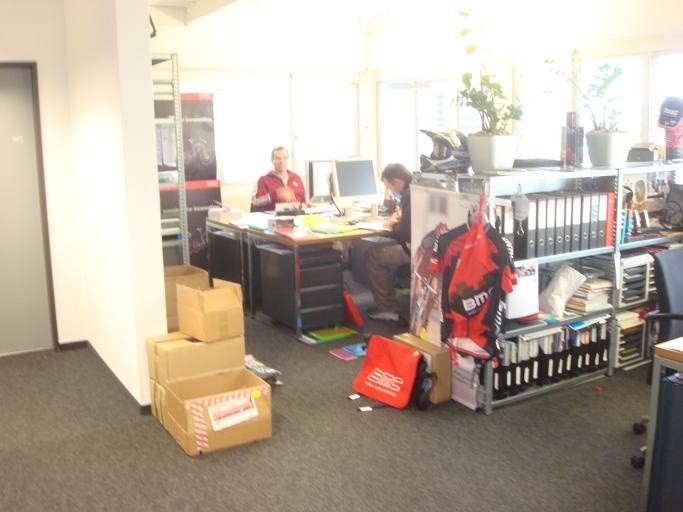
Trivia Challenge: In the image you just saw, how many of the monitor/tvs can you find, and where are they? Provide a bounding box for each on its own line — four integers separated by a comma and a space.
309, 159, 379, 217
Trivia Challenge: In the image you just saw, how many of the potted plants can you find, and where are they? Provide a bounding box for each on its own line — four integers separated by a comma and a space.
583, 61, 622, 168
455, 70, 522, 175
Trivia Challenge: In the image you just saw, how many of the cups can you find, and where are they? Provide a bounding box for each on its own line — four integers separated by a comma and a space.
370, 203, 379, 217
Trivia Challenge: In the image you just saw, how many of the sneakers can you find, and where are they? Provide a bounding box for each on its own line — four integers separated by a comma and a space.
365, 305, 400, 320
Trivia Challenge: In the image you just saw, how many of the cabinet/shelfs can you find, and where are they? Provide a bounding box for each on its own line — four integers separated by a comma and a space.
149, 52, 189, 267
411, 161, 683, 416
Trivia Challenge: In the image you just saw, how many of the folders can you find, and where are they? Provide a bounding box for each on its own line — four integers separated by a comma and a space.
492, 193, 616, 259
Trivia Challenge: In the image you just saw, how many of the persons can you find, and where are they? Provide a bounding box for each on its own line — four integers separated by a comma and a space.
249, 146, 317, 211
364, 162, 414, 321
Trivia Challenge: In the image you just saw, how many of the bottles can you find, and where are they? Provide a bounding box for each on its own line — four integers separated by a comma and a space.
559, 112, 585, 171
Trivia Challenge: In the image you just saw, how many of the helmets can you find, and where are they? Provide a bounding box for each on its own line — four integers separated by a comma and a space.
419, 129, 470, 173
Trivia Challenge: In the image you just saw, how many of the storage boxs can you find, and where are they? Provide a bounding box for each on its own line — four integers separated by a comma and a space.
145, 264, 271, 457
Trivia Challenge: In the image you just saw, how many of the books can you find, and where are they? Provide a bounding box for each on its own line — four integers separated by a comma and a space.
311, 223, 360, 234
500, 262, 613, 367
620, 208, 683, 269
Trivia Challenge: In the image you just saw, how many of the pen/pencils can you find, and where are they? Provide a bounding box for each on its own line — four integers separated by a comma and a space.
292, 202, 304, 210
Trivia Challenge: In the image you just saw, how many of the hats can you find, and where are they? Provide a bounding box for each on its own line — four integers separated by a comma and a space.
659, 97, 683, 126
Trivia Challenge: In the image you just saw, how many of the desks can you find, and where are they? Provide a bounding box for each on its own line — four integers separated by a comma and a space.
637, 336, 683, 512
206, 198, 404, 337
